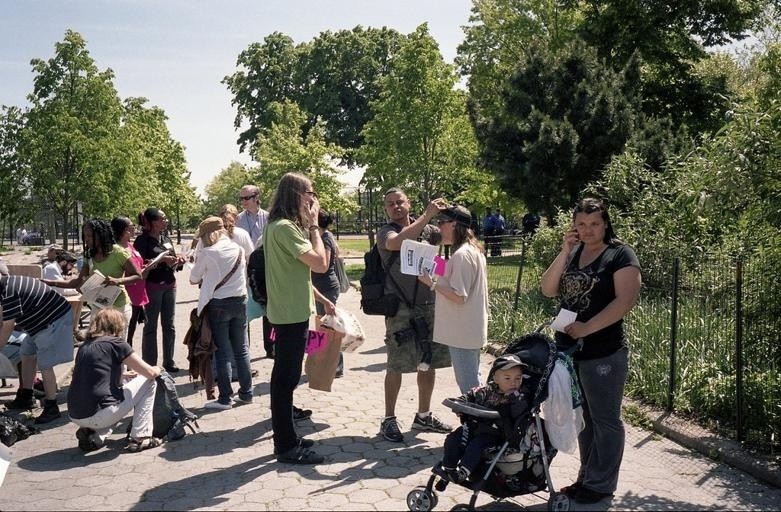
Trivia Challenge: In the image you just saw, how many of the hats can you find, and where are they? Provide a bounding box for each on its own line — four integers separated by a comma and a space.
435, 203, 474, 229
492, 353, 528, 372
195, 216, 224, 239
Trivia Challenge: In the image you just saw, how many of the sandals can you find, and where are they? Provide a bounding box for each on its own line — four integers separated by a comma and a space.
126, 436, 164, 453
76, 428, 99, 451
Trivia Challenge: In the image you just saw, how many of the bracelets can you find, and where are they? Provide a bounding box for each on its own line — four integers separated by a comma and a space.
429, 275, 438, 291
308, 224, 318, 230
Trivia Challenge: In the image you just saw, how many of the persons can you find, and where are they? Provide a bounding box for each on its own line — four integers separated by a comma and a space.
1, 266, 76, 423
134, 208, 185, 372
15, 225, 27, 246
255, 236, 275, 358
79, 218, 140, 341
369, 188, 453, 442
436, 353, 532, 480
482, 207, 498, 251
219, 203, 255, 316
419, 204, 488, 397
305, 206, 344, 379
236, 185, 268, 321
43, 257, 67, 286
69, 310, 161, 452
522, 212, 539, 238
264, 174, 335, 464
493, 209, 506, 255
542, 197, 641, 504
191, 219, 252, 410
110, 216, 157, 352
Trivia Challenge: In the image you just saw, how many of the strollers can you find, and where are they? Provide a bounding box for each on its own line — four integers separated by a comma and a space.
407, 322, 583, 512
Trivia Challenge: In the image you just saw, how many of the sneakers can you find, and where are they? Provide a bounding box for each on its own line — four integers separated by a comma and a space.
204, 399, 233, 410
560, 482, 579, 497
233, 395, 252, 405
251, 370, 258, 377
379, 416, 405, 444
3, 389, 38, 410
434, 477, 449, 492
447, 468, 467, 485
411, 412, 455, 435
33, 399, 61, 425
570, 487, 611, 503
297, 433, 315, 447
293, 404, 313, 420
275, 449, 325, 465
164, 362, 179, 374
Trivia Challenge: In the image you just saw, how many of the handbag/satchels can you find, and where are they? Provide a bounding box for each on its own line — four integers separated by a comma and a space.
304, 307, 344, 392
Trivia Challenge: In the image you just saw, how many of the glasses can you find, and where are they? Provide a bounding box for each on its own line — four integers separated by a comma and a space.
130, 224, 137, 227
305, 190, 319, 197
239, 193, 256, 202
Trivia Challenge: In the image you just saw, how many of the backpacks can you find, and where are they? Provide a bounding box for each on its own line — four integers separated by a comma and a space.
360, 223, 400, 316
247, 245, 267, 307
128, 363, 196, 439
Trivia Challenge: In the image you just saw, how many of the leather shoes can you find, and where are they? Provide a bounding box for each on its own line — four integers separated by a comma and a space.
267, 349, 275, 357
